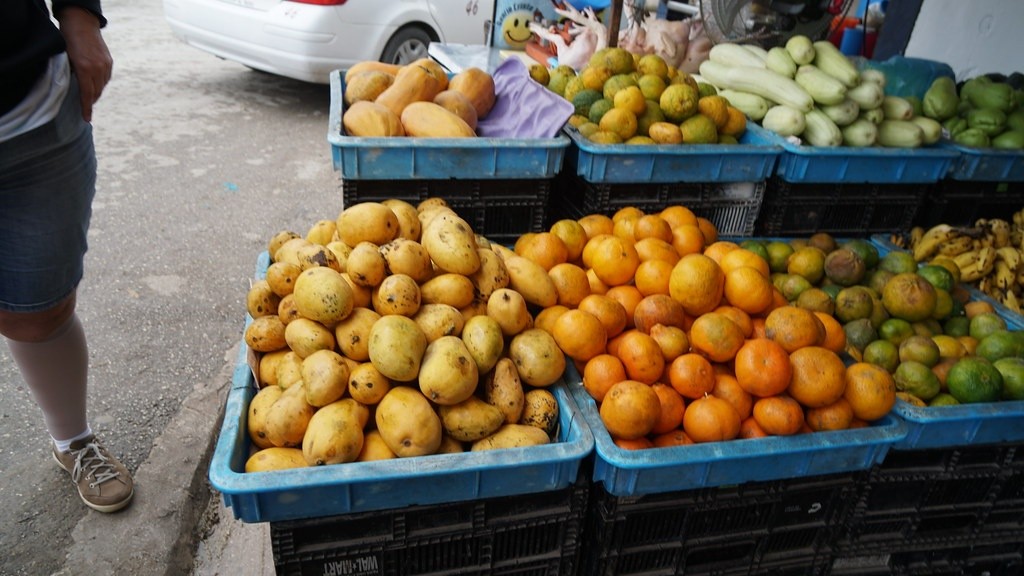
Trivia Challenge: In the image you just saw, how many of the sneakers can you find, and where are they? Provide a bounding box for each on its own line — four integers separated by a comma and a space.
48, 432, 135, 515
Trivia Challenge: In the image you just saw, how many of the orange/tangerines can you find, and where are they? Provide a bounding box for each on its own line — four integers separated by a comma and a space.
515, 207, 1024, 448
530, 43, 745, 150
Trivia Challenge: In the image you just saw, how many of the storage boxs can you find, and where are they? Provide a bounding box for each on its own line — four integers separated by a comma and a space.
206, 67, 1024, 576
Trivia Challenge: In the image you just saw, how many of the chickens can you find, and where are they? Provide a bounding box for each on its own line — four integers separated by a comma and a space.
529, 0, 715, 78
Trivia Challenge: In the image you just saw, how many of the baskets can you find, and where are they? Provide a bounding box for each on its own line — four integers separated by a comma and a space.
256, 441, 1024, 576
338, 176, 1023, 247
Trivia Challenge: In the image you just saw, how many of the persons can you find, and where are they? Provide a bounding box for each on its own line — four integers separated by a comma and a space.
0, 0, 134, 513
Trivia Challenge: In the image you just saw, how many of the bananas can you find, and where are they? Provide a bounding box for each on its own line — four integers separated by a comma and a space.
893, 212, 1020, 312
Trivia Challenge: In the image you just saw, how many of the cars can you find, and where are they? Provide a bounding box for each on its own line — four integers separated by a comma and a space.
164, 1, 495, 86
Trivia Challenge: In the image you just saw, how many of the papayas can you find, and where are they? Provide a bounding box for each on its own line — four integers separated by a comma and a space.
338, 56, 499, 149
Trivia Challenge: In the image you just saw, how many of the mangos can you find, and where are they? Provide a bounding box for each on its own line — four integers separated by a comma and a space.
243, 199, 563, 471
